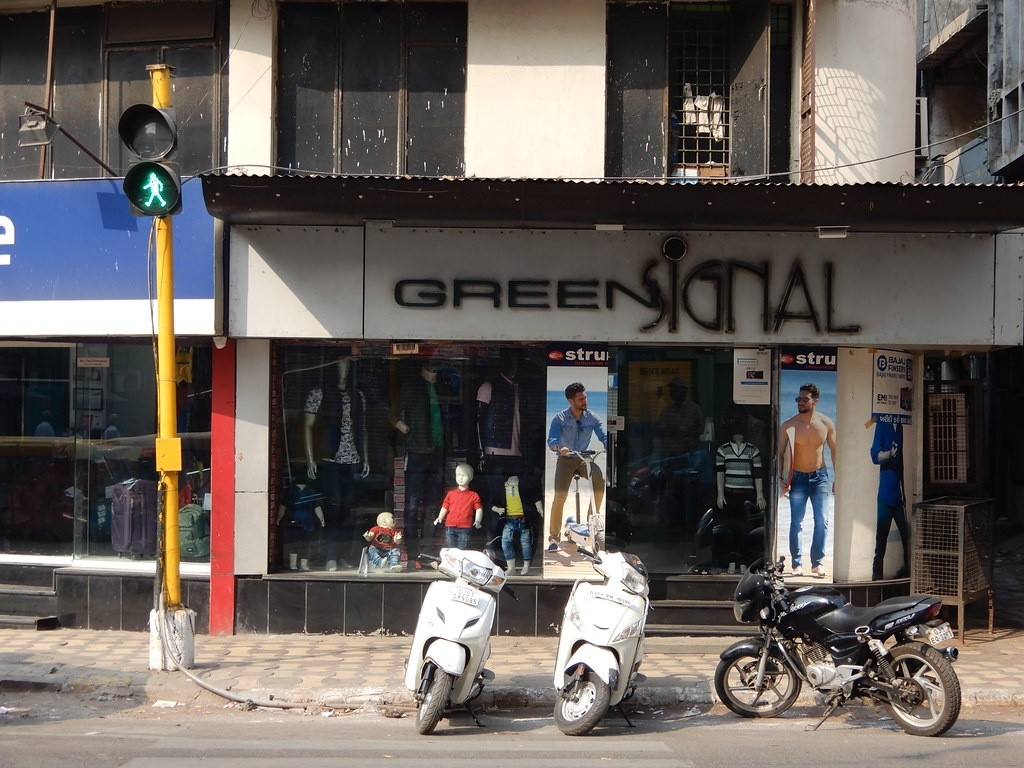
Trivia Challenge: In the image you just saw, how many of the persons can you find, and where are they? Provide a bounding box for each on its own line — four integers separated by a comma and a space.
29, 410, 55, 470
548, 382, 607, 552
363, 512, 403, 573
303, 356, 370, 572
870, 420, 908, 580
473, 347, 536, 542
433, 461, 483, 549
487, 475, 544, 575
654, 374, 704, 544
101, 412, 120, 440
715, 434, 766, 574
779, 383, 837, 577
19, 451, 104, 539
385, 365, 453, 571
277, 484, 324, 572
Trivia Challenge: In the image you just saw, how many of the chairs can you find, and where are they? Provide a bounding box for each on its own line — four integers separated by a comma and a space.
358, 547, 370, 573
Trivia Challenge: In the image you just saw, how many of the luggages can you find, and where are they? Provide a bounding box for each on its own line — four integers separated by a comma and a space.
111, 479, 157, 559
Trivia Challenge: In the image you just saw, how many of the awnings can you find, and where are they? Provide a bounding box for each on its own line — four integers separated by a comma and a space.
199, 172, 1024, 235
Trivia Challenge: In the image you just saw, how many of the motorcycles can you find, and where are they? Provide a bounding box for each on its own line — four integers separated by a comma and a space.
714, 555, 962, 736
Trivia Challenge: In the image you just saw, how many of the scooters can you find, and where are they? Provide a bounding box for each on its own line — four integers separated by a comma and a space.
552, 513, 661, 735
402, 534, 522, 736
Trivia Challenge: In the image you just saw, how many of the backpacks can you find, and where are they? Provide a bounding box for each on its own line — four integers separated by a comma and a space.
180, 504, 210, 557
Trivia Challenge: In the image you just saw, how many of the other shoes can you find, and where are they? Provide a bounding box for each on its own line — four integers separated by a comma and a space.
548, 543, 557, 552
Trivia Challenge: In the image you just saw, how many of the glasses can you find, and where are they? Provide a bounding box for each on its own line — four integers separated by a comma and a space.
796, 397, 814, 402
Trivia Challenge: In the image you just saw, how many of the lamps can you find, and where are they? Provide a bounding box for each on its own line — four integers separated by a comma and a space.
814, 225, 850, 239
18, 101, 118, 177
362, 219, 396, 228
593, 224, 626, 231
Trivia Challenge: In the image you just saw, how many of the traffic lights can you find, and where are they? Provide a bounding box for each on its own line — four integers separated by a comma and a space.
116, 102, 181, 219
